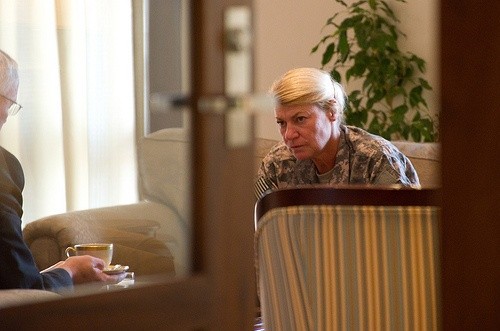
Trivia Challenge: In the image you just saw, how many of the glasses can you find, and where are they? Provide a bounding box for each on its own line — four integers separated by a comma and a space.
0, 95, 22, 114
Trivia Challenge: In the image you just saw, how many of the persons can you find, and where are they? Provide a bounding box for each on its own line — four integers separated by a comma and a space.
0, 50, 128, 291
255, 68, 423, 201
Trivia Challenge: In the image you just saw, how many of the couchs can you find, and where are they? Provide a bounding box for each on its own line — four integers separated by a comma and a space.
0, 127, 438, 331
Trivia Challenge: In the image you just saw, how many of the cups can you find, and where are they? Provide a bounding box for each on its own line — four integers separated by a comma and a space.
66, 244, 113, 266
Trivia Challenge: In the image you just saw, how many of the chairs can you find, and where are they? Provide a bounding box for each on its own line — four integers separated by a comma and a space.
251, 186, 442, 331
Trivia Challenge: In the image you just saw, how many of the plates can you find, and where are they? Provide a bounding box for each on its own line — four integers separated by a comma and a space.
103, 266, 129, 275
